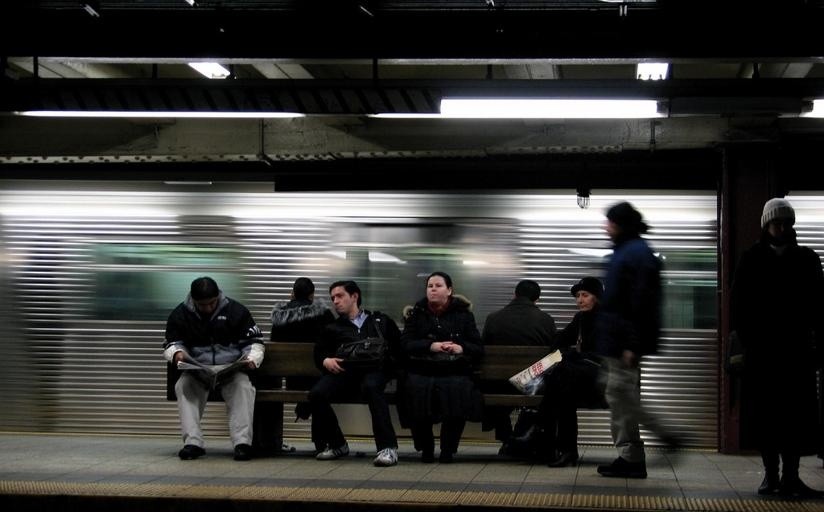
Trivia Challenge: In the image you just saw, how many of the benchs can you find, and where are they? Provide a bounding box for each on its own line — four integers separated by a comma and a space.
166, 341, 608, 468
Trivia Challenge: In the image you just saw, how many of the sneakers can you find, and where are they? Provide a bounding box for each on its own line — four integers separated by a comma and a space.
176, 440, 207, 461
595, 450, 649, 480
310, 439, 352, 460
419, 445, 436, 465
438, 445, 457, 466
232, 440, 254, 462
371, 446, 402, 467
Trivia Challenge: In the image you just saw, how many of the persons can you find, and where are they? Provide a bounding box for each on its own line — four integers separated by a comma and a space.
270, 276, 335, 451
727, 198, 824, 499
515, 276, 611, 468
163, 277, 263, 461
308, 279, 401, 466
483, 279, 555, 453
597, 202, 687, 477
396, 272, 481, 462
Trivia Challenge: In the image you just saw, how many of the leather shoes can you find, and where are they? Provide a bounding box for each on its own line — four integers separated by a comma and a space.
757, 467, 781, 496
548, 444, 579, 469
778, 471, 824, 503
496, 424, 537, 459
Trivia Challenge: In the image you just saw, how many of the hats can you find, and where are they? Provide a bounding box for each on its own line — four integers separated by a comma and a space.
570, 274, 606, 301
606, 199, 650, 234
758, 196, 798, 232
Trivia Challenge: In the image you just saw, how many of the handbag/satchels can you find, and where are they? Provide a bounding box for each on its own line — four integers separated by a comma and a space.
404, 333, 465, 367
507, 348, 564, 396
330, 336, 401, 373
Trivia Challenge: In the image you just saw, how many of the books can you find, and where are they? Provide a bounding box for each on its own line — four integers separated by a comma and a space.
175, 350, 251, 393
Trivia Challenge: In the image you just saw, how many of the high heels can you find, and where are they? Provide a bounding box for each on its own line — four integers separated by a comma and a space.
293, 406, 311, 424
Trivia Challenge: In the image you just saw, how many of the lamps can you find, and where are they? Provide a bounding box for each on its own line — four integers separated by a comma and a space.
636, 62, 669, 81
575, 182, 592, 210
186, 61, 231, 80
438, 93, 669, 122
